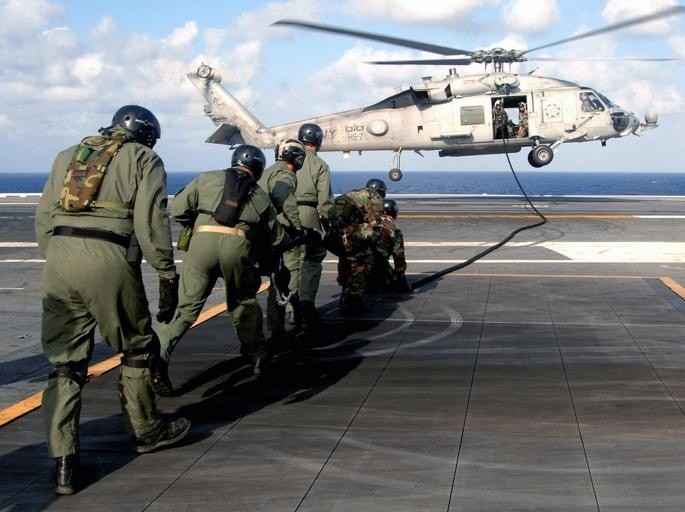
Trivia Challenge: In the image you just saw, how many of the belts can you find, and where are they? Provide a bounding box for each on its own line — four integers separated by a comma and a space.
195, 224, 248, 237
296, 201, 317, 207
52, 226, 130, 248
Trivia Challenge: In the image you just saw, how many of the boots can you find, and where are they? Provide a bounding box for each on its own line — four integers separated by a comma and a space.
344, 294, 376, 313
135, 417, 192, 454
149, 357, 175, 396
55, 456, 75, 494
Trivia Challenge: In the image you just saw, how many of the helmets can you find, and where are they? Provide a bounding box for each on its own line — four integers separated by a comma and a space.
111, 105, 160, 149
299, 123, 325, 149
366, 179, 387, 197
232, 144, 267, 179
274, 139, 305, 169
383, 199, 398, 219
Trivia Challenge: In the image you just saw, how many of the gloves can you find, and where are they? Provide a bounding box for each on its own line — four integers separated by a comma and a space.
156, 273, 180, 325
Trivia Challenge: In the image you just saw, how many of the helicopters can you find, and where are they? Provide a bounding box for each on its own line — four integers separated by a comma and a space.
174, 0, 684, 181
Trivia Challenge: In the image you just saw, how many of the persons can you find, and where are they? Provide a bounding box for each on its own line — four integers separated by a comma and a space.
492, 97, 528, 141
34, 105, 191, 495
257, 123, 412, 341
151, 143, 295, 395
